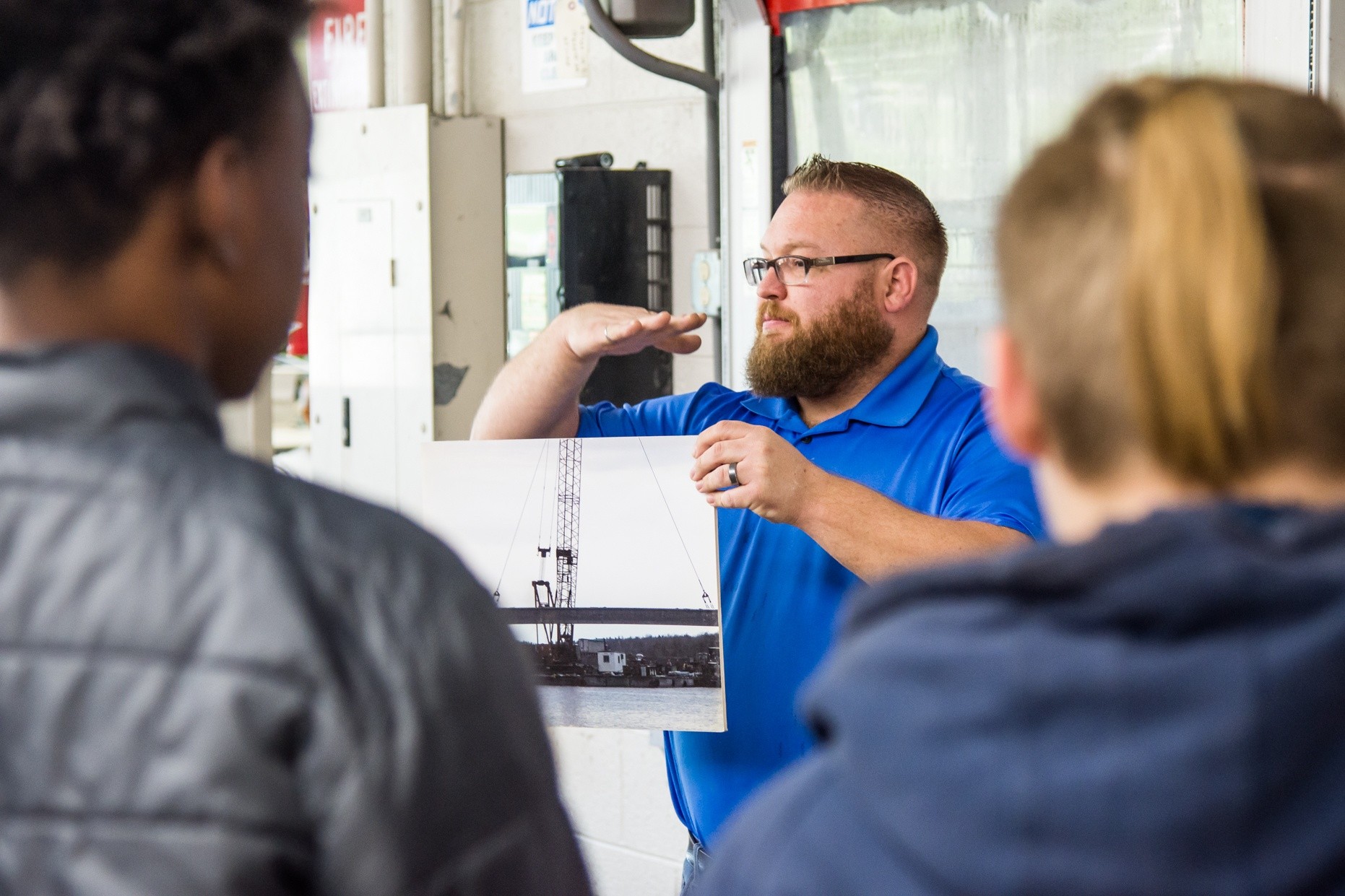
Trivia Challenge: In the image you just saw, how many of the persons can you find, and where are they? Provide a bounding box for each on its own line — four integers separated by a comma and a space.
684, 80, 1345, 896
0, 0, 593, 895
469, 146, 1049, 880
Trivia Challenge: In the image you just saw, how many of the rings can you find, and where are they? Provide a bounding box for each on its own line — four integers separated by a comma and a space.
729, 463, 739, 487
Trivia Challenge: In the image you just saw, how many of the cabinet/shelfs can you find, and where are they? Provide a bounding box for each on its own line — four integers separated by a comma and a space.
308, 103, 507, 449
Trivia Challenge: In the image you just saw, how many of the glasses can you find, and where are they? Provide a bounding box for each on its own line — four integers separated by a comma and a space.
743, 251, 895, 285
